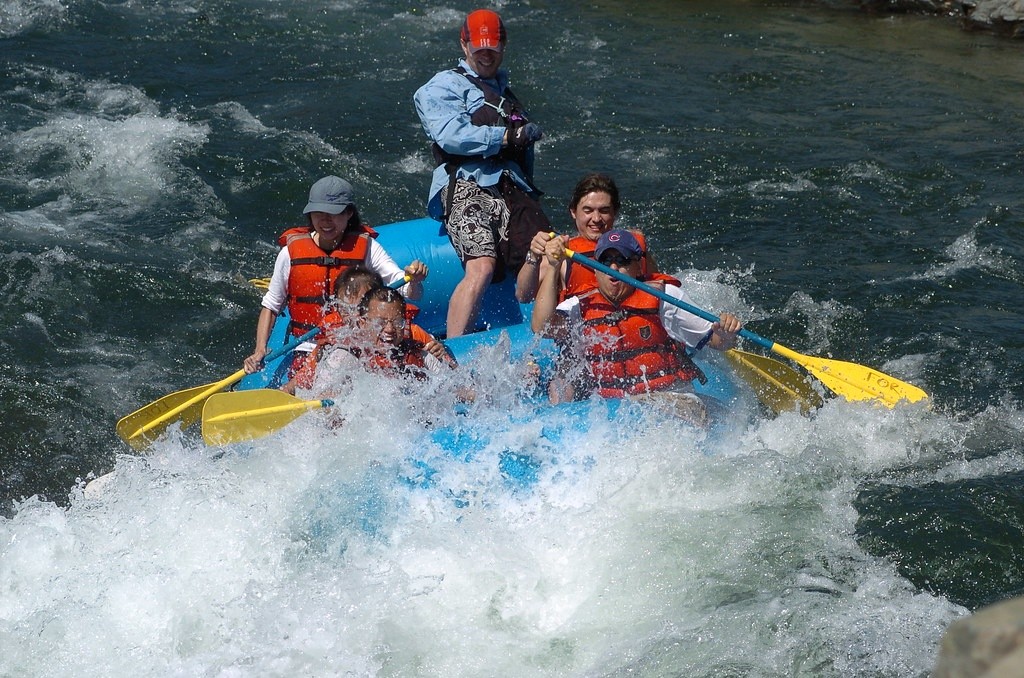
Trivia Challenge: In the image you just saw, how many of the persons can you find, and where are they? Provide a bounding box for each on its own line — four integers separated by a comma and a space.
413, 8, 559, 340
244, 172, 739, 433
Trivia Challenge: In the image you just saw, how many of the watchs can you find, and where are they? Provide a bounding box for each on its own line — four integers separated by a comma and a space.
525, 251, 543, 266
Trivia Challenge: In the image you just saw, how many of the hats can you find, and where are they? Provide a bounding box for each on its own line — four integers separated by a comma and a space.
461, 9, 507, 55
596, 229, 642, 261
303, 175, 355, 214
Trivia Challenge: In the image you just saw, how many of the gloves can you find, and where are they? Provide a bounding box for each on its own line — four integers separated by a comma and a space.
507, 122, 542, 151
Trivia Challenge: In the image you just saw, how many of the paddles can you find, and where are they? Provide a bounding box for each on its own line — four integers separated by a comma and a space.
543, 227, 823, 409
562, 250, 934, 409
202, 377, 346, 448
117, 272, 415, 460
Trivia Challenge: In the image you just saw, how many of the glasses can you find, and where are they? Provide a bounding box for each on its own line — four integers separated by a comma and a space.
363, 317, 406, 331
599, 253, 631, 268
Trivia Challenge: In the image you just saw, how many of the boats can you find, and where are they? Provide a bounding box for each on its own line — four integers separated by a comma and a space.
238, 211, 747, 536
